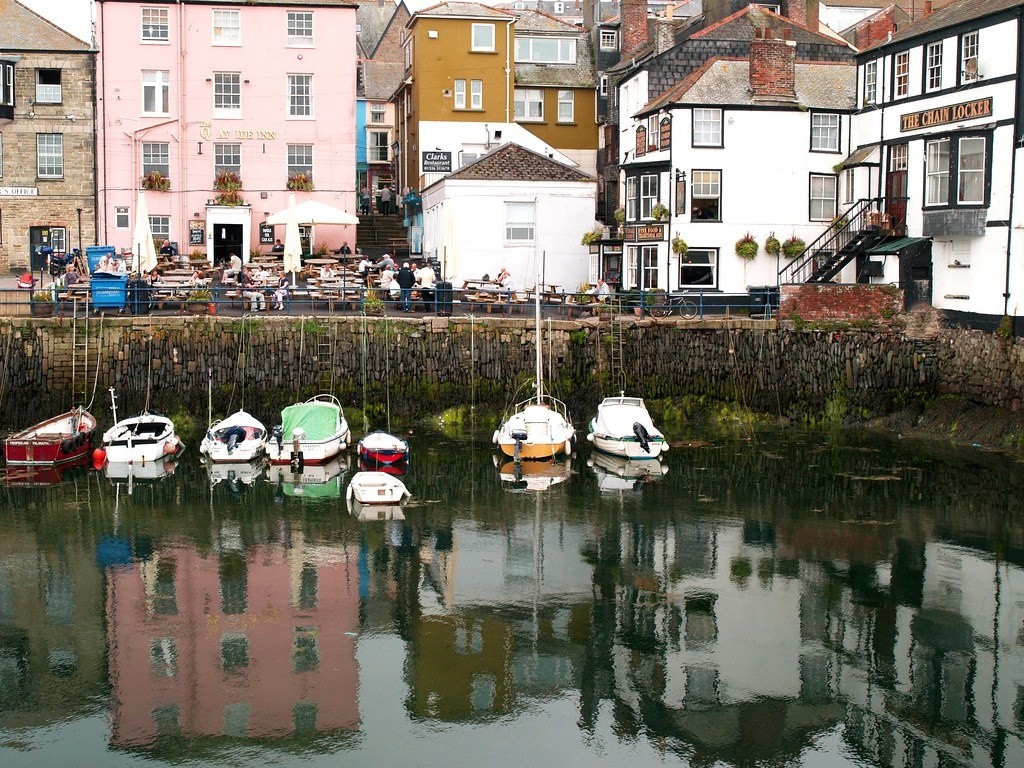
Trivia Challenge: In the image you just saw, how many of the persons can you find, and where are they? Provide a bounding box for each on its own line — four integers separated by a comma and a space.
100, 253, 114, 270
238, 266, 248, 283
397, 262, 416, 313
272, 271, 290, 310
359, 255, 372, 276
149, 269, 165, 293
594, 278, 611, 302
65, 264, 81, 288
380, 185, 391, 215
242, 271, 268, 311
142, 274, 148, 279
271, 239, 285, 253
416, 263, 436, 312
188, 271, 207, 286
360, 183, 371, 215
376, 252, 402, 296
254, 266, 272, 277
410, 263, 422, 285
322, 265, 334, 278
496, 266, 512, 290
160, 240, 177, 261
340, 242, 352, 254
220, 253, 242, 284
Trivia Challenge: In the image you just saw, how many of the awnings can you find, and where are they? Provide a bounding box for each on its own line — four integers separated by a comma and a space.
866, 238, 926, 251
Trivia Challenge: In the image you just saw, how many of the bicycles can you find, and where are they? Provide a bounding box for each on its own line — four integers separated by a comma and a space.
650, 289, 697, 320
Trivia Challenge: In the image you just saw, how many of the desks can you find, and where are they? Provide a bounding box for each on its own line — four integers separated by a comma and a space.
66, 237, 561, 308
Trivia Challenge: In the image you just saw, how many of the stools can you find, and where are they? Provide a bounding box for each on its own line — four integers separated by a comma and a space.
58, 260, 576, 317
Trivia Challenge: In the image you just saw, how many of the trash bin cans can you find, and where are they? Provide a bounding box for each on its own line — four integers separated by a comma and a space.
766, 286, 780, 310
89, 274, 129, 314
436, 282, 453, 316
86, 246, 116, 274
130, 280, 149, 314
747, 286, 767, 316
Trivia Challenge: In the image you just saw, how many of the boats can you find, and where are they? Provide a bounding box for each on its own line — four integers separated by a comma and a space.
347, 471, 410, 504
201, 464, 267, 494
493, 394, 576, 460
493, 459, 570, 495
352, 499, 406, 522
200, 408, 268, 464
587, 390, 669, 460
102, 410, 185, 464
104, 463, 188, 495
0, 452, 91, 489
262, 456, 351, 503
3, 405, 97, 467
264, 394, 351, 465
586, 448, 670, 507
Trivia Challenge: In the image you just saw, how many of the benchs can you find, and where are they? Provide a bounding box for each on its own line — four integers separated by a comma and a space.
39, 433, 72, 440
388, 243, 409, 257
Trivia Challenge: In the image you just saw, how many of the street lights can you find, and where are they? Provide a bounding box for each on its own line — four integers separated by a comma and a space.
76, 206, 83, 249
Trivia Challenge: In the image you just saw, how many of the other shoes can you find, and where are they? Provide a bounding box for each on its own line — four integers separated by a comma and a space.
407, 309, 415, 313
279, 305, 284, 310
260, 307, 267, 311
274, 304, 279, 308
251, 308, 258, 312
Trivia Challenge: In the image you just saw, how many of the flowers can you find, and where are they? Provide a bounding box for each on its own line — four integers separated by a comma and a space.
214, 191, 243, 206
215, 172, 243, 192
142, 171, 170, 191
735, 231, 758, 260
287, 172, 314, 192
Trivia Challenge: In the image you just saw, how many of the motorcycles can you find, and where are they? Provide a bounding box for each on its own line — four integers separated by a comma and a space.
15, 272, 39, 294
41, 247, 81, 276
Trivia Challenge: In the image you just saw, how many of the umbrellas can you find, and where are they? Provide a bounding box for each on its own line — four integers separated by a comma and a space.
283, 193, 303, 285
131, 191, 158, 274
266, 199, 360, 255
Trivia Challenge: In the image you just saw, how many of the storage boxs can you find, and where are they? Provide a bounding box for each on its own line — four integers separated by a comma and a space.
866, 212, 892, 229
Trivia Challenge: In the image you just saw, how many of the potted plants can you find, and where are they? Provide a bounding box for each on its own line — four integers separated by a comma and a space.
598, 304, 619, 321
362, 298, 385, 317
187, 289, 213, 315
32, 289, 53, 318
782, 238, 805, 257
581, 232, 603, 246
